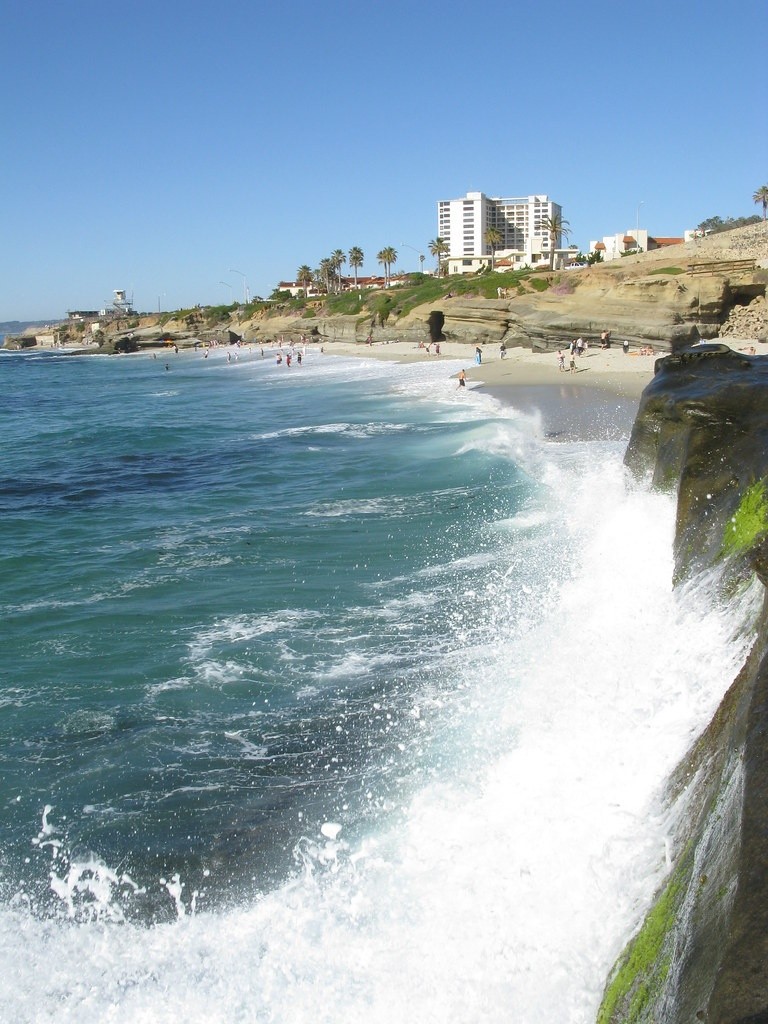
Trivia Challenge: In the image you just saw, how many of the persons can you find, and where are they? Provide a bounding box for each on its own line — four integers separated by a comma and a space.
748, 346, 758, 357
476, 347, 482, 364
153, 334, 325, 371
456, 369, 466, 390
556, 330, 653, 373
500, 343, 506, 358
418, 341, 440, 357
497, 285, 507, 299
